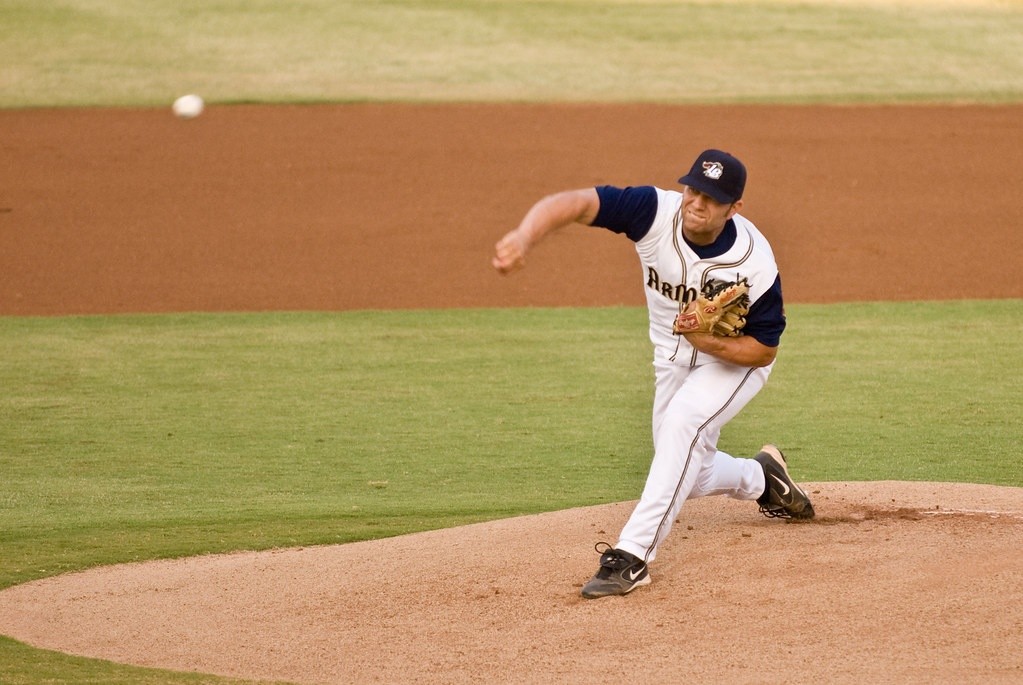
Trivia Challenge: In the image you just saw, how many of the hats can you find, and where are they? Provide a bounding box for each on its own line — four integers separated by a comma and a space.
677, 149, 747, 203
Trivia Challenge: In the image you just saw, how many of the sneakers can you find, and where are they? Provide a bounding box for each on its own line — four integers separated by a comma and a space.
581, 542, 652, 598
752, 442, 815, 519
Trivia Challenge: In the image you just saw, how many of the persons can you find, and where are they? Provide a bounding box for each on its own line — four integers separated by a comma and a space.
495, 149, 815, 600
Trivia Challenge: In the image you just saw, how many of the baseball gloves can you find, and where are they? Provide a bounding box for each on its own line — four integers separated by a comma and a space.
673, 278, 751, 338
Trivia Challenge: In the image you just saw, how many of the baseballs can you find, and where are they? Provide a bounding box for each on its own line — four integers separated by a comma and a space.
172, 95, 204, 120
